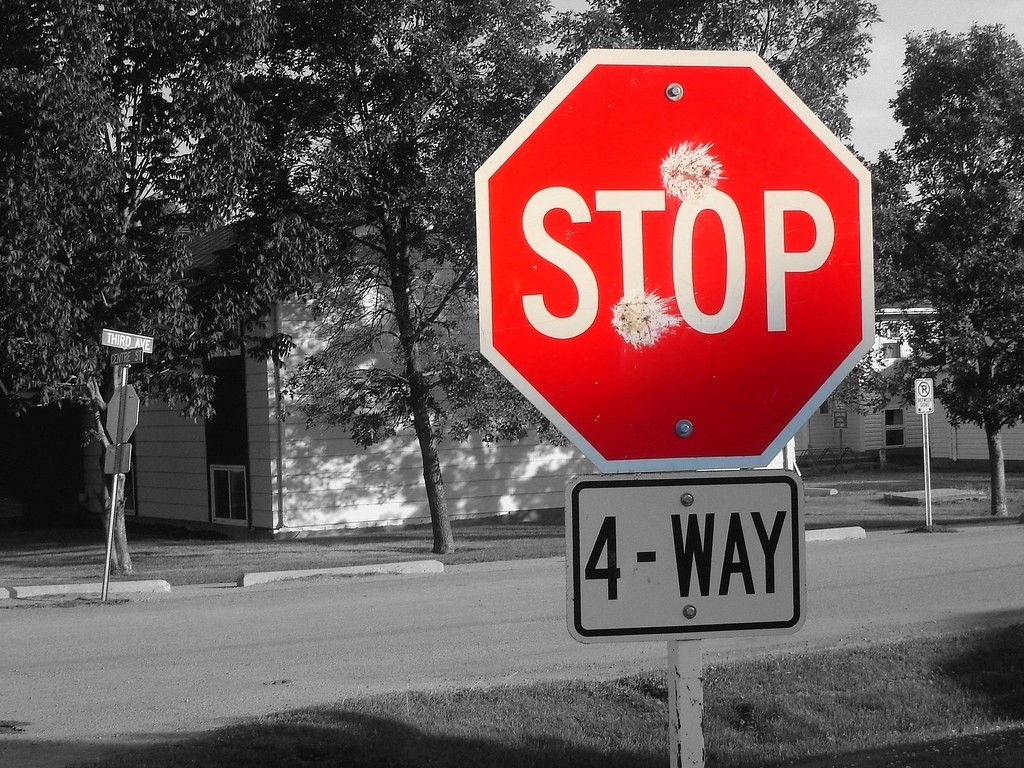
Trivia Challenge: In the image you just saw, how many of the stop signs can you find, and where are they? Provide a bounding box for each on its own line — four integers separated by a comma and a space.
466, 50, 883, 469
